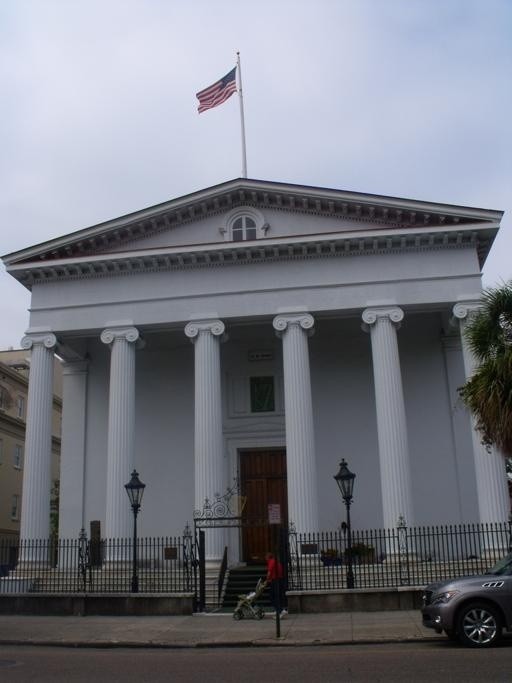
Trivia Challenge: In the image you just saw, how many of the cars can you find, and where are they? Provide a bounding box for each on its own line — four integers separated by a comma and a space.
422, 551, 512, 648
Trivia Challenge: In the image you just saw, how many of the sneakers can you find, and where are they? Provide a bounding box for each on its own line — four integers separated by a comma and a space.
272, 609, 288, 620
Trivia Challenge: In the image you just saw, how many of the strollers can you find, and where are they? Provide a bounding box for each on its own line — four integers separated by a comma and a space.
232, 576, 268, 621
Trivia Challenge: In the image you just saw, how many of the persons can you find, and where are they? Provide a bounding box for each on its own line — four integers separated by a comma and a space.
263, 548, 289, 621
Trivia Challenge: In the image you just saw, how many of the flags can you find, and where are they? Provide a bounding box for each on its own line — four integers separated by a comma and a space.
195, 67, 237, 113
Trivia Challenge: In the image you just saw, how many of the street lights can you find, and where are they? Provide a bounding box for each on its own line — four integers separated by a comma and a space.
125, 469, 146, 593
333, 458, 356, 589
